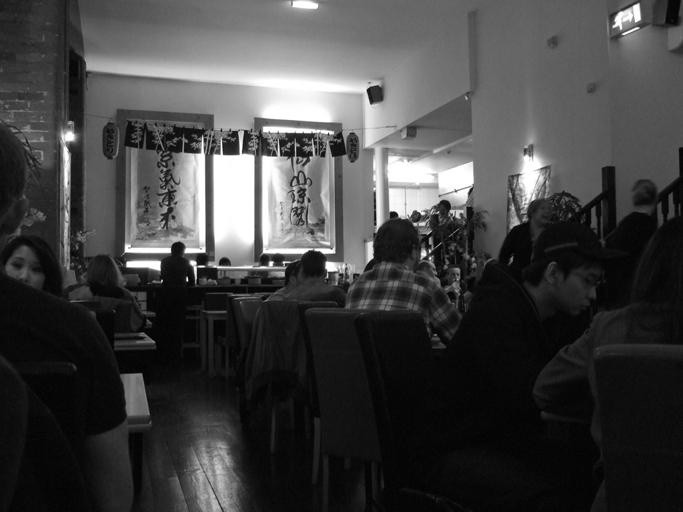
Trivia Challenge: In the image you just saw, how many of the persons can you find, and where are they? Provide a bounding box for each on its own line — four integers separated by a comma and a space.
598, 179, 657, 314
160, 241, 194, 289
432, 200, 681, 510
266, 200, 476, 352
247, 254, 269, 279
218, 256, 236, 280
64, 254, 146, 333
196, 253, 216, 284
1, 125, 135, 511
2, 234, 63, 298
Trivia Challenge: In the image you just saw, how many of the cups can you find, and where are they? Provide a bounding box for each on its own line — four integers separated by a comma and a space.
328, 271, 339, 286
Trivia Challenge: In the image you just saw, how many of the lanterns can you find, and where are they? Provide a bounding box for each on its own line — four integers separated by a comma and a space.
347, 132, 359, 163
102, 122, 118, 160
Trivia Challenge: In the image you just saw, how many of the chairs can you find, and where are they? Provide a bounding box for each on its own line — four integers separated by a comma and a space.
0, 283, 683, 512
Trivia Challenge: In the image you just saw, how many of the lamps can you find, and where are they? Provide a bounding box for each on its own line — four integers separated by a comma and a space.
291, 0, 319, 12
521, 145, 532, 160
65, 119, 75, 145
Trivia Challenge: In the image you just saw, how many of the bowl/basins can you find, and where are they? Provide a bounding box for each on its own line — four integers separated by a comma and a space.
199, 278, 218, 286
248, 275, 263, 285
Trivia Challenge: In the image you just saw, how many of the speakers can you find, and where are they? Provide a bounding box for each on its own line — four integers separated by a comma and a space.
367, 85, 383, 104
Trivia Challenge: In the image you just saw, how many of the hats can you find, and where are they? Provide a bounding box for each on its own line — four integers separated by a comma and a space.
518, 218, 617, 278
